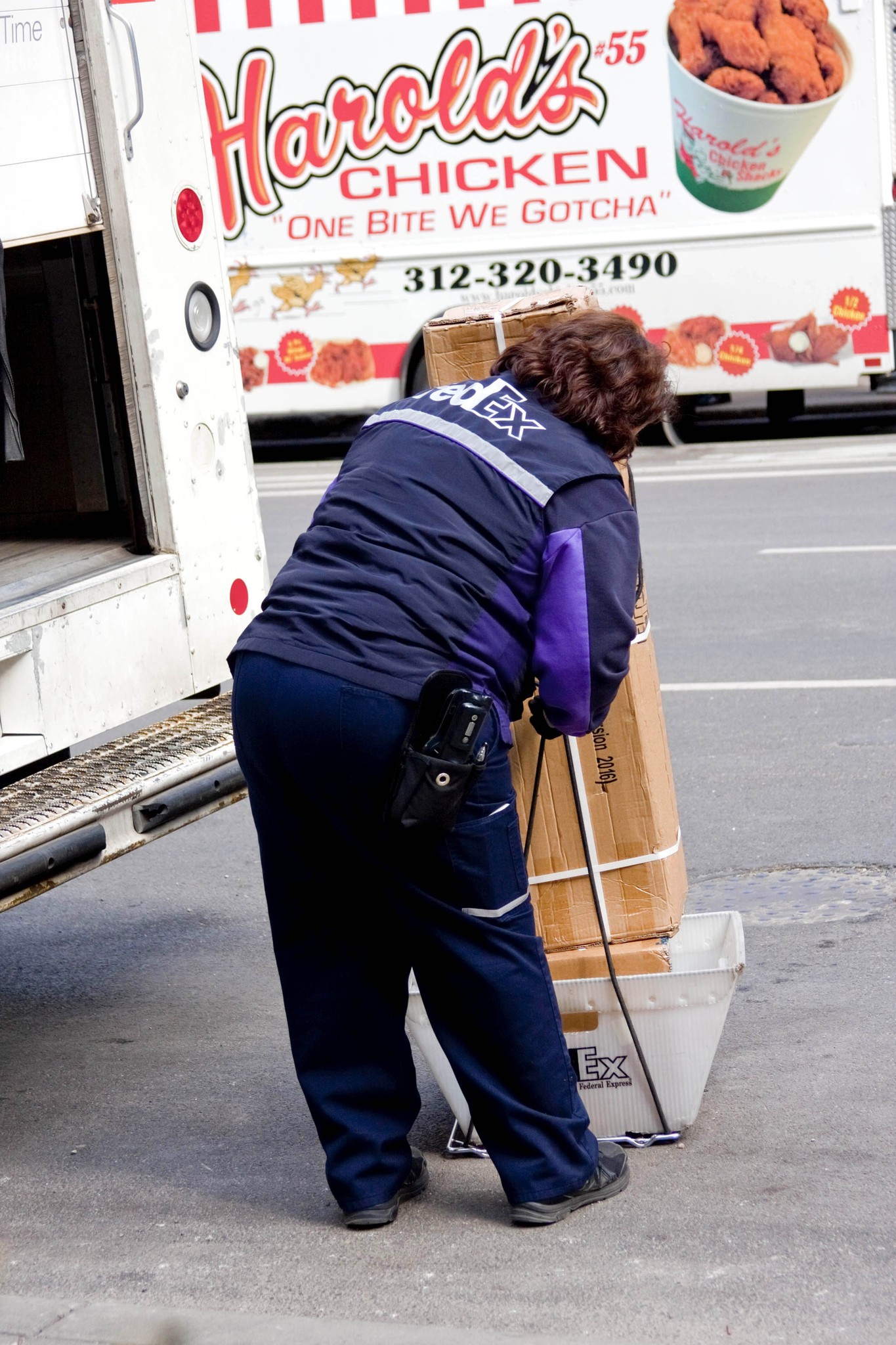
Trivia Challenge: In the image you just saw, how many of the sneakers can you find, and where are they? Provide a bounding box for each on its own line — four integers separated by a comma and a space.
345, 1144, 427, 1227
511, 1140, 629, 1222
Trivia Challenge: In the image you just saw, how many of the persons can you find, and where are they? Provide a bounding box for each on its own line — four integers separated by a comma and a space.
227, 311, 674, 1229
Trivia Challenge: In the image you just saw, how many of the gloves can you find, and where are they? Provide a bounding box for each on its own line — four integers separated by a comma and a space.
528, 694, 563, 739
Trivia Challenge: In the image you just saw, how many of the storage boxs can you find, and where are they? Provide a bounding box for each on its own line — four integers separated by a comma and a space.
421, 288, 687, 950
404, 909, 746, 1146
538, 937, 673, 981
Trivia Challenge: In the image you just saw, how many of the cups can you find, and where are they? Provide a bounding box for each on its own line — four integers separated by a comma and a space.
662, 10, 856, 214
